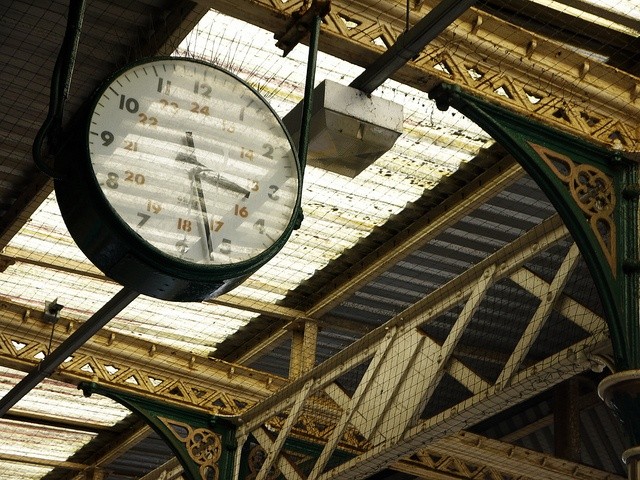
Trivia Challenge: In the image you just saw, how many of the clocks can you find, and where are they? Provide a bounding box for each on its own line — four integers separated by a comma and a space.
55, 56, 303, 301
